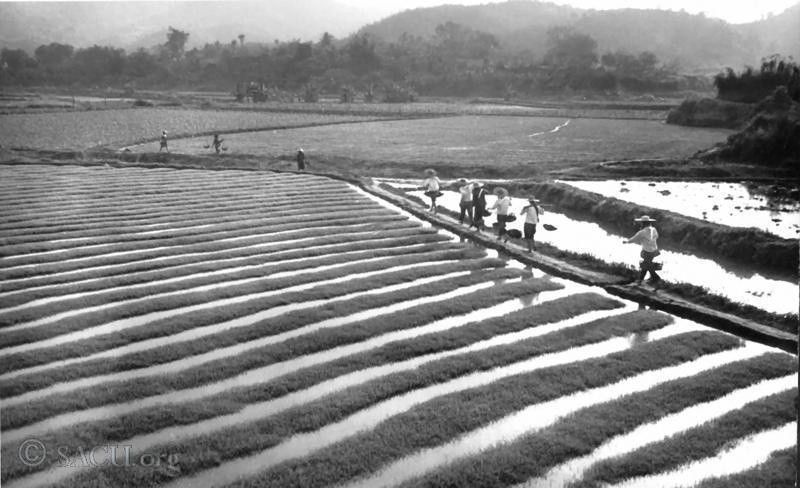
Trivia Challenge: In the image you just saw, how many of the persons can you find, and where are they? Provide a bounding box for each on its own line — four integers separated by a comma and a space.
296, 148, 305, 171
520, 194, 544, 253
212, 134, 222, 153
469, 182, 487, 231
488, 187, 513, 243
623, 216, 664, 291
423, 168, 443, 214
455, 178, 474, 226
159, 129, 171, 154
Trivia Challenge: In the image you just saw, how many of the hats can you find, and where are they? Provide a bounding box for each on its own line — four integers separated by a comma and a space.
633, 215, 657, 221
424, 168, 437, 177
525, 194, 540, 202
493, 186, 509, 196
456, 178, 484, 189
